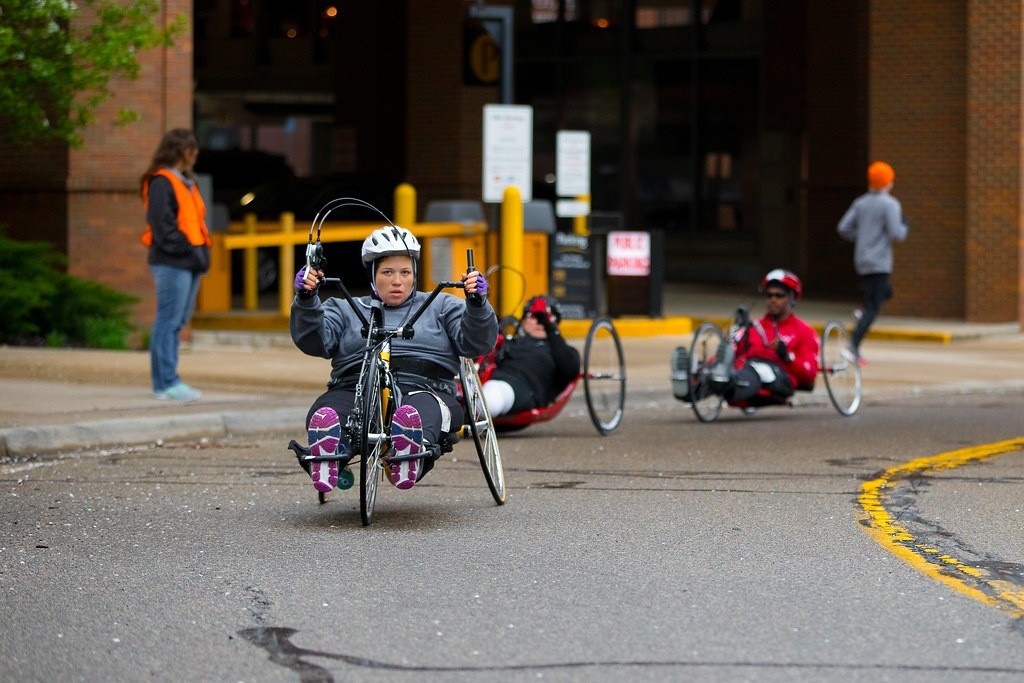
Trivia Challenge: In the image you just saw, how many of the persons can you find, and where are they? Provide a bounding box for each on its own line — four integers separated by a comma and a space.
291, 225, 499, 492
454, 295, 581, 443
672, 268, 821, 411
139, 128, 212, 400
836, 161, 909, 366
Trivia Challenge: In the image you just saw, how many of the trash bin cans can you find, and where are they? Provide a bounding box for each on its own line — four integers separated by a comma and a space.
422, 198, 488, 299
605, 229, 666, 318
488, 199, 557, 336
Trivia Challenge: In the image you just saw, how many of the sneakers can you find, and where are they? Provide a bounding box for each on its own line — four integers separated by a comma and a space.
843, 342, 872, 368
671, 346, 690, 397
854, 309, 871, 334
711, 337, 733, 382
308, 406, 342, 493
391, 405, 423, 490
152, 383, 202, 402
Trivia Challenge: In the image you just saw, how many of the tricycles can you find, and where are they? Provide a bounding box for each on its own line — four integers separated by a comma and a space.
286, 197, 508, 525
463, 266, 628, 435
686, 307, 865, 423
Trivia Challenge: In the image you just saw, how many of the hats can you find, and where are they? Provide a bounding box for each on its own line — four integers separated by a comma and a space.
867, 161, 895, 187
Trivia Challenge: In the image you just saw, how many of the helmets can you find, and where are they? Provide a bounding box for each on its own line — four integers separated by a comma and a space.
524, 296, 562, 325
362, 225, 421, 268
763, 269, 802, 307
